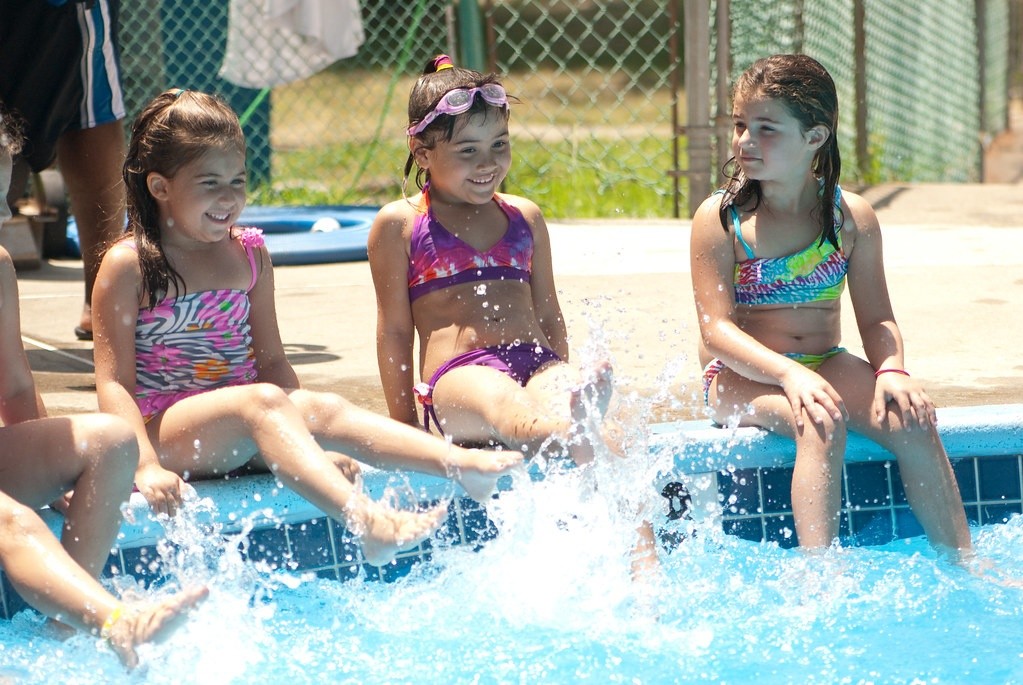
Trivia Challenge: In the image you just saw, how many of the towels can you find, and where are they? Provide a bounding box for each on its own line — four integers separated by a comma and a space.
218, 0, 366, 89
75, 0, 127, 130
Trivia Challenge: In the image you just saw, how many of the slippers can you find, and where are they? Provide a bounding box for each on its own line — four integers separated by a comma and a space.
75, 326, 94, 340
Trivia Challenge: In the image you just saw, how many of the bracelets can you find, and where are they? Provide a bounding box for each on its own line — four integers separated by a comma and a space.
874, 368, 910, 379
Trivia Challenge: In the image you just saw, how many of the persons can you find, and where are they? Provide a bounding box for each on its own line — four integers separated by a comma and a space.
92, 88, 525, 567
0, 115, 211, 671
367, 55, 660, 576
690, 52, 972, 557
0, 0, 130, 339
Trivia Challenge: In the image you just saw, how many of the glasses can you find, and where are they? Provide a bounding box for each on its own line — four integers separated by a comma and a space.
409, 84, 509, 136
